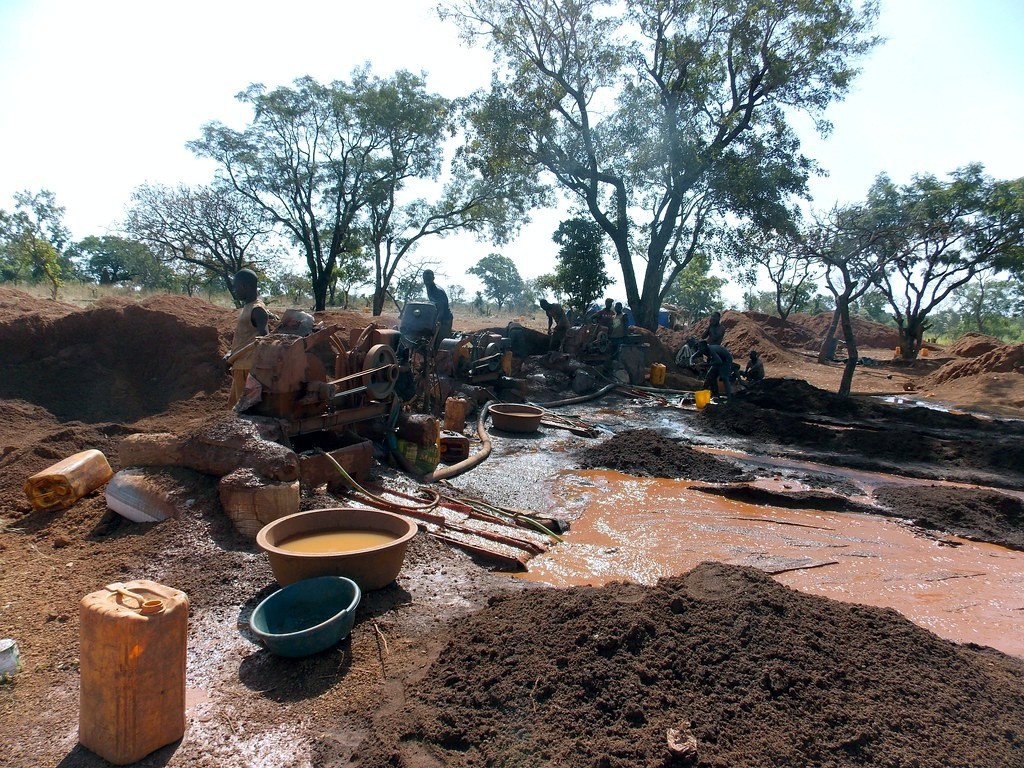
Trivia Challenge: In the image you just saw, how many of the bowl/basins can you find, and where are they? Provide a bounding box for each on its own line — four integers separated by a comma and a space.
488, 403, 545, 433
248, 577, 361, 658
256, 508, 419, 595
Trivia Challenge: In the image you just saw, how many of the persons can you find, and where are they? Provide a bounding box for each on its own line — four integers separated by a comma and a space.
689, 340, 733, 403
540, 299, 570, 351
423, 269, 453, 357
608, 302, 628, 360
676, 337, 700, 376
590, 299, 614, 340
222, 269, 269, 410
701, 312, 725, 345
742, 351, 765, 384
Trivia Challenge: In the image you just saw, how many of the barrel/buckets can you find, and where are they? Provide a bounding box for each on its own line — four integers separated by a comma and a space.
443, 395, 468, 434
694, 389, 711, 408
387, 408, 440, 473
78, 579, 189, 767
23, 449, 114, 516
440, 430, 469, 464
821, 337, 837, 359
650, 362, 666, 385
921, 348, 928, 357
896, 347, 900, 354
